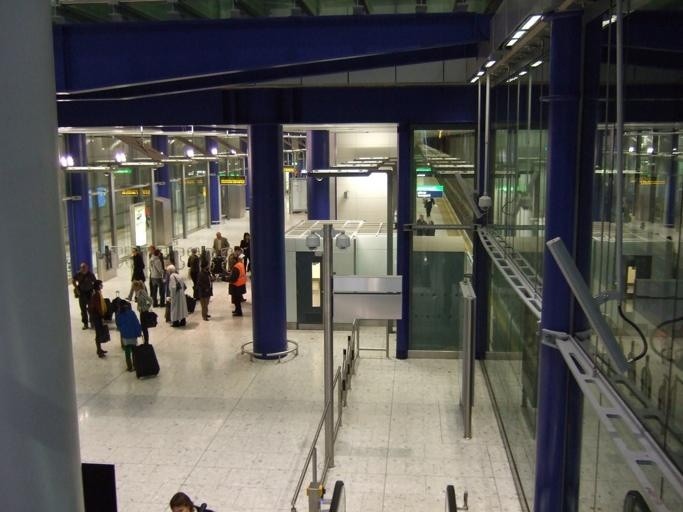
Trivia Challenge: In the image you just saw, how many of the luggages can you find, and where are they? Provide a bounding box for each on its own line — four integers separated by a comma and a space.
129, 333, 160, 380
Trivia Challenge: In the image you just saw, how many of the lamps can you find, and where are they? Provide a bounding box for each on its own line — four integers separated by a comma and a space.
469, 16, 548, 84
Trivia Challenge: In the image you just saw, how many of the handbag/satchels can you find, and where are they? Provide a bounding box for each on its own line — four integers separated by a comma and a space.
171, 273, 182, 291
140, 304, 158, 329
96, 323, 111, 344
72, 285, 80, 298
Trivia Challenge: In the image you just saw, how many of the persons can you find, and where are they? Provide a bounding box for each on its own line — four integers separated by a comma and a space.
416, 196, 435, 236
72, 232, 250, 372
171, 491, 214, 512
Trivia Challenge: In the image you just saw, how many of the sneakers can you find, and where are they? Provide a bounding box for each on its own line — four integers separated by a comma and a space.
169, 307, 248, 329
96, 349, 108, 358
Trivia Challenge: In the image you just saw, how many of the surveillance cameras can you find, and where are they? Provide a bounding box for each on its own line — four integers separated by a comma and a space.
478, 197, 492, 211
336, 234, 350, 249
306, 233, 319, 250
